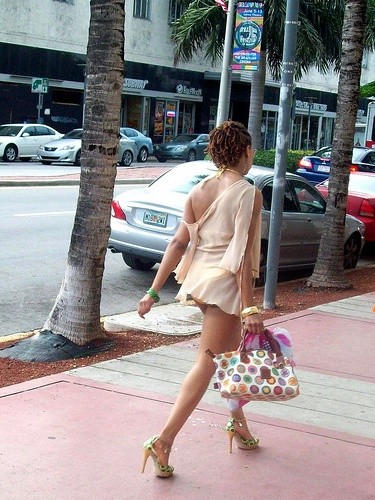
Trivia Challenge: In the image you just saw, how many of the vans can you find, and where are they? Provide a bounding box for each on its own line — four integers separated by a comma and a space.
364, 102, 375, 148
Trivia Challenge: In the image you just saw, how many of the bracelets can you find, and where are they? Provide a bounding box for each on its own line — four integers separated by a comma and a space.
241, 305, 258, 318
147, 288, 159, 302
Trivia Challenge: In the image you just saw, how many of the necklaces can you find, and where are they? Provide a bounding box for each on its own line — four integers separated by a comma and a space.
215, 164, 240, 178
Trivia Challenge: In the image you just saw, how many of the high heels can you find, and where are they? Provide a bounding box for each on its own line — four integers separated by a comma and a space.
227, 418, 260, 453
142, 435, 174, 477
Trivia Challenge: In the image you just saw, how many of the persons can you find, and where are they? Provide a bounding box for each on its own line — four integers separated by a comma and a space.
138, 119, 265, 477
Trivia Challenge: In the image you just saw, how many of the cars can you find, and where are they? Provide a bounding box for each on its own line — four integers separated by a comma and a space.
109, 160, 366, 286
119, 127, 154, 162
155, 133, 212, 163
0, 124, 66, 163
296, 146, 375, 185
296, 168, 375, 249
37, 128, 137, 168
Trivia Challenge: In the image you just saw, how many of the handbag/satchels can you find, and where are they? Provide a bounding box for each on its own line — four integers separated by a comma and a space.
228, 326, 294, 412
206, 328, 300, 402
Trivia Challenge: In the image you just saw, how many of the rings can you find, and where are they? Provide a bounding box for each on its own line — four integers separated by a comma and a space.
261, 329, 265, 331
